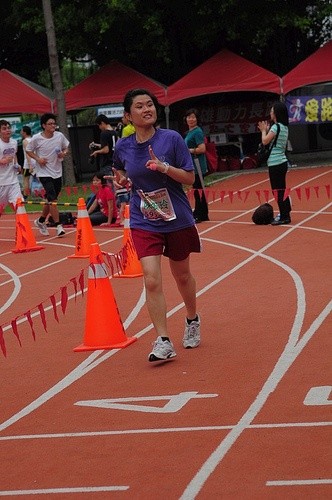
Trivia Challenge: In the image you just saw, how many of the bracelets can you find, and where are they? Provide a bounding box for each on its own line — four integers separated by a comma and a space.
64, 152, 66, 153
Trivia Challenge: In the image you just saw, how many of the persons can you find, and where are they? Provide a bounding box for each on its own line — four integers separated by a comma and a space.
103, 88, 202, 360
116, 112, 135, 141
89, 114, 116, 191
0, 120, 25, 215
21, 126, 36, 196
26, 113, 70, 236
184, 108, 209, 223
258, 101, 292, 225
87, 172, 118, 226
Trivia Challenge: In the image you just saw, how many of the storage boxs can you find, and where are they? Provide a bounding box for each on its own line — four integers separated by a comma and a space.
209, 133, 228, 143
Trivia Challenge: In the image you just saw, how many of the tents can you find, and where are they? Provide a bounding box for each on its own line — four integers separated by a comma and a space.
0, 68, 55, 120
167, 49, 283, 110
54, 62, 169, 129
283, 39, 332, 94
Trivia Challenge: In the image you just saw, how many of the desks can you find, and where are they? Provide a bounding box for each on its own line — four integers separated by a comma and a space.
213, 142, 244, 157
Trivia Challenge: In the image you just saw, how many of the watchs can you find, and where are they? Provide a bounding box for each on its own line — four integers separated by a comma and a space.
162, 162, 169, 173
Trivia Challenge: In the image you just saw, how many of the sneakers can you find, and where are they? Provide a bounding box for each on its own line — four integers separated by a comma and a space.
148, 335, 177, 361
55, 224, 66, 236
272, 214, 292, 225
35, 218, 50, 236
181, 313, 201, 349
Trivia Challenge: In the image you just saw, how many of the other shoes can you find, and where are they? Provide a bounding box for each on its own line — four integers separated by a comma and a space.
194, 216, 209, 223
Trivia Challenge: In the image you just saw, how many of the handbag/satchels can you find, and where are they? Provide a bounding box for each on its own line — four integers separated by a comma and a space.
258, 123, 279, 162
205, 140, 218, 172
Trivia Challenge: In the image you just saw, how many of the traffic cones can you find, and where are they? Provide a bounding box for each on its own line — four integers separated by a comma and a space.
11, 197, 45, 253
67, 197, 98, 259
113, 204, 145, 279
72, 242, 139, 353
